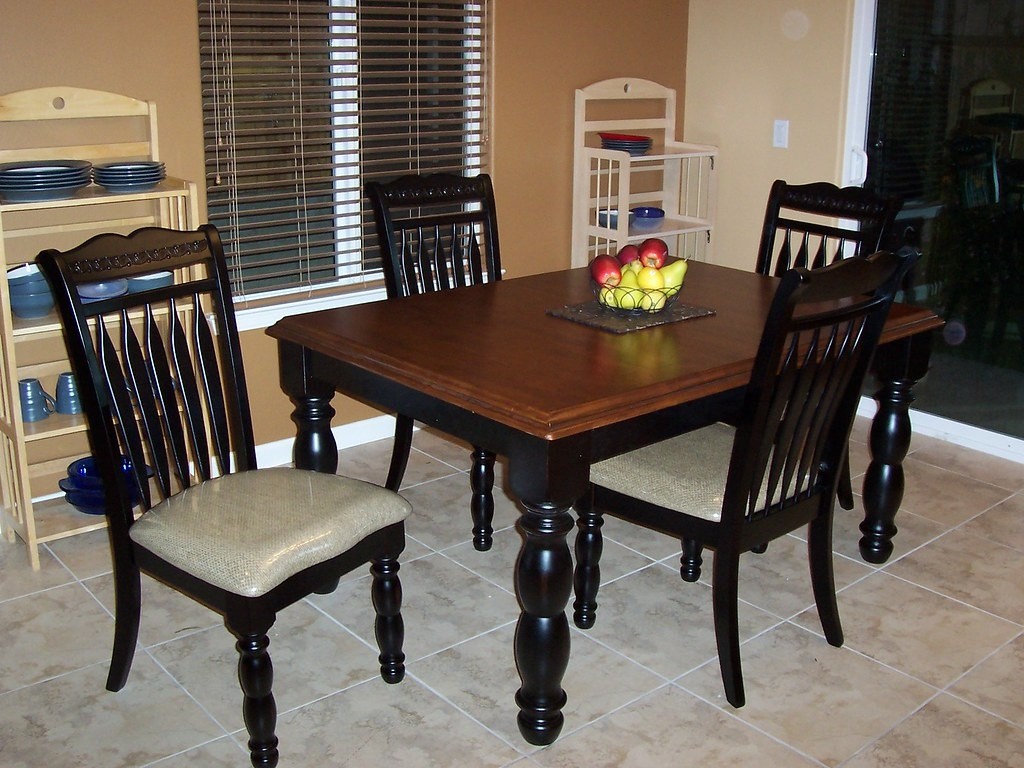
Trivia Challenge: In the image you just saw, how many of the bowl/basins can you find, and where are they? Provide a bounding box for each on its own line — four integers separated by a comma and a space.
8, 262, 174, 317
58, 454, 156, 516
595, 209, 634, 229
630, 207, 665, 231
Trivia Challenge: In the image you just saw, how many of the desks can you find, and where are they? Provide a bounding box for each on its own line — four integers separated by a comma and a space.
263, 255, 945, 748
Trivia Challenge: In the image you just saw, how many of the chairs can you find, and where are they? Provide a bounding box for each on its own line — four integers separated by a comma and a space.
35, 224, 413, 768
570, 243, 922, 707
725, 179, 906, 555
366, 175, 503, 552
967, 79, 1024, 159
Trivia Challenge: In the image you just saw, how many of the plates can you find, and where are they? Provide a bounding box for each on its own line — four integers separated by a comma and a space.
0, 158, 168, 204
596, 132, 654, 157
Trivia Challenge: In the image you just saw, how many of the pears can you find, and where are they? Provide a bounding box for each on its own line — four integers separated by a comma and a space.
600, 260, 687, 313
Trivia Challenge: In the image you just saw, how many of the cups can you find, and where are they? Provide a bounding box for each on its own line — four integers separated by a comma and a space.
110, 357, 178, 417
18, 378, 58, 424
56, 371, 84, 416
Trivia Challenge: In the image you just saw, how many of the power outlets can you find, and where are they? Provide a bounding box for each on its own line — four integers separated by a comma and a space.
772, 120, 789, 149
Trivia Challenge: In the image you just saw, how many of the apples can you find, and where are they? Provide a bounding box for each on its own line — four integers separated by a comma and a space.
590, 238, 668, 286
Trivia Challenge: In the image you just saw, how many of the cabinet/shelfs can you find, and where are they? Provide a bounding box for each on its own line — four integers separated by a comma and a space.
0, 86, 213, 571
568, 77, 718, 263
